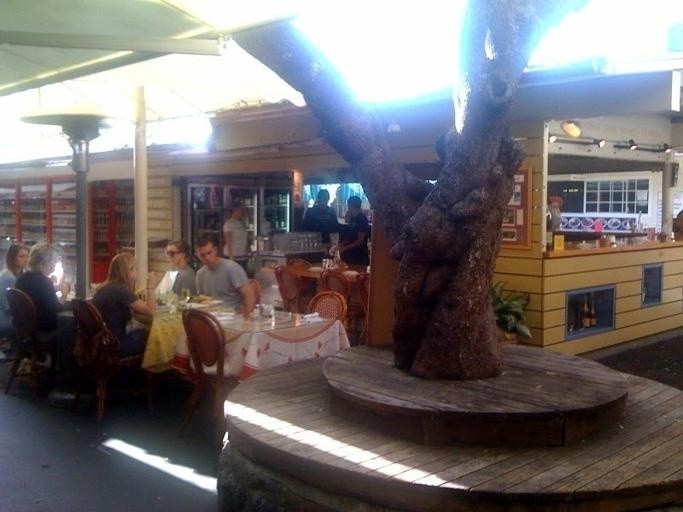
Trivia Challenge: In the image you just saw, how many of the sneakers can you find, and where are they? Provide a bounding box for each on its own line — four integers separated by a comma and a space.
1, 348, 16, 362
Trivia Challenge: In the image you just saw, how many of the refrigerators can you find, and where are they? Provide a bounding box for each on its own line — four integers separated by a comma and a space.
178, 175, 292, 276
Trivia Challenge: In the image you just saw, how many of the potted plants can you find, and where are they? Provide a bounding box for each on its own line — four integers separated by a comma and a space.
490, 281, 533, 345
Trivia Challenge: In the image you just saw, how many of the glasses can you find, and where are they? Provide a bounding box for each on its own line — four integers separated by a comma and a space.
166, 248, 183, 257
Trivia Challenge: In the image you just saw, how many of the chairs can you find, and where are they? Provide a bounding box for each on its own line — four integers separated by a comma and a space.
179, 309, 230, 445
99, 258, 369, 324
3, 287, 67, 398
72, 299, 154, 423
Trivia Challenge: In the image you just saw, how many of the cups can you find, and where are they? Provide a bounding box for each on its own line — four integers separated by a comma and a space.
646, 227, 655, 240
265, 231, 324, 255
666, 231, 675, 244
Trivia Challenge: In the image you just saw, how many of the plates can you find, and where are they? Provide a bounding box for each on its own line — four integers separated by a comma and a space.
138, 287, 325, 325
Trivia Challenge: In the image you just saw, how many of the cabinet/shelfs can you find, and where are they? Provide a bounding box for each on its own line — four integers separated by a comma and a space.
187, 184, 291, 256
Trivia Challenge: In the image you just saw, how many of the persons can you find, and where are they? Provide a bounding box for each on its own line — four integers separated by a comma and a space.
333, 196, 369, 266
304, 189, 337, 259
222, 203, 248, 272
4, 233, 254, 375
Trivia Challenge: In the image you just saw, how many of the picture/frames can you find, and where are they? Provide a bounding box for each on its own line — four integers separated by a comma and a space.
496, 164, 533, 250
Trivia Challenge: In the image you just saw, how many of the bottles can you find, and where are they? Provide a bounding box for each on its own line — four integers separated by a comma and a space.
308, 248, 372, 278
590, 300, 598, 328
607, 235, 616, 245
599, 239, 609, 246
581, 296, 590, 328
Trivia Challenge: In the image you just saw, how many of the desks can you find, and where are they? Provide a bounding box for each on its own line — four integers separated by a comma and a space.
155, 299, 349, 379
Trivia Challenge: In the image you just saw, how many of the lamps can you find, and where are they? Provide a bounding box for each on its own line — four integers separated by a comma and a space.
560, 119, 582, 139
548, 133, 606, 149
614, 139, 671, 153
